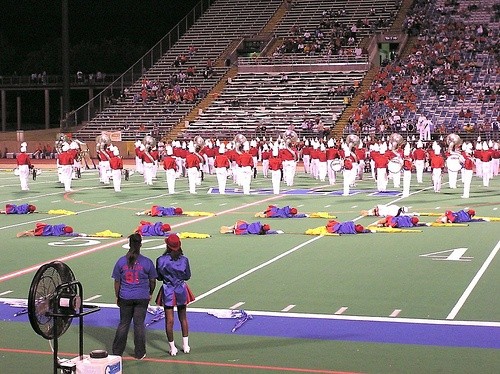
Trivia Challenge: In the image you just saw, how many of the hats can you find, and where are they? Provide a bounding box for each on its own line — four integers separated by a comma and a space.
165, 234, 181, 251
290, 208, 298, 214
402, 206, 408, 212
411, 218, 419, 225
175, 208, 182, 215
263, 223, 270, 231
468, 209, 476, 215
17, 136, 499, 156
355, 225, 364, 232
161, 224, 171, 231
29, 205, 36, 211
64, 226, 73, 233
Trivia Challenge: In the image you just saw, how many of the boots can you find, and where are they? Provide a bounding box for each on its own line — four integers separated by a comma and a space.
181, 336, 191, 355
167, 340, 178, 357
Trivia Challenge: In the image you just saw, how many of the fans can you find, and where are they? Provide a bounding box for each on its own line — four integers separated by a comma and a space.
14, 261, 101, 374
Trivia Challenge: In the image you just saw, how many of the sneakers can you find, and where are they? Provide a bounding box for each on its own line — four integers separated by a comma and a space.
136, 352, 146, 360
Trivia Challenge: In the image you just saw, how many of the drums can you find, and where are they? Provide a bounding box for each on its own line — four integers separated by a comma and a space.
330, 158, 343, 173
387, 158, 403, 174
445, 154, 464, 171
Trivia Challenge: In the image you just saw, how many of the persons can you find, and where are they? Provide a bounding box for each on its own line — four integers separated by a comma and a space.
0, 203, 37, 215
325, 220, 372, 234
156, 232, 191, 357
132, 220, 177, 237
16, 222, 84, 238
111, 232, 157, 361
15, 0, 500, 198
136, 205, 183, 216
219, 219, 278, 236
367, 215, 426, 229
435, 209, 486, 224
359, 204, 408, 218
254, 205, 310, 219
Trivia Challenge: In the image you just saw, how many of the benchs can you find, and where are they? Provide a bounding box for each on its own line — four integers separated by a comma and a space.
60, 0, 500, 145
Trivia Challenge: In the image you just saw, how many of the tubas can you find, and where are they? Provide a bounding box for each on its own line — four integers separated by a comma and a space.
56, 135, 81, 191
95, 135, 99, 152
100, 135, 110, 161
192, 135, 206, 166
346, 135, 361, 164
446, 132, 462, 156
282, 129, 298, 161
390, 133, 403, 157
143, 136, 155, 164
235, 133, 246, 156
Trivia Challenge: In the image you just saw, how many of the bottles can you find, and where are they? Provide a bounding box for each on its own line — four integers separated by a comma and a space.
76, 349, 122, 374
